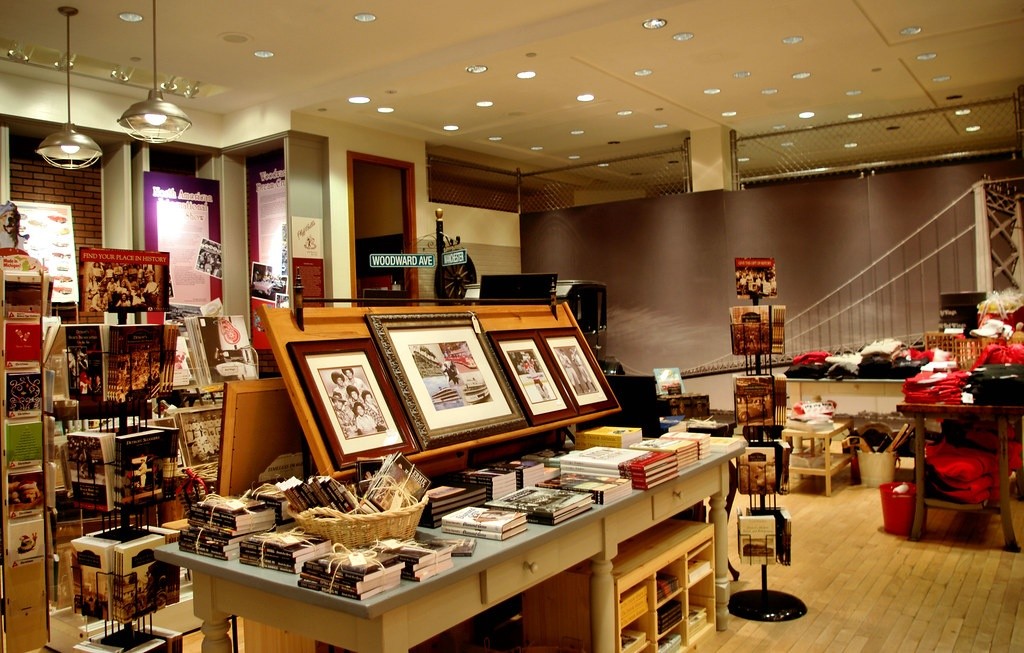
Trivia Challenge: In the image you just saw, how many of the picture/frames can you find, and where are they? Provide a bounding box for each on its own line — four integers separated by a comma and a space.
284, 312, 620, 472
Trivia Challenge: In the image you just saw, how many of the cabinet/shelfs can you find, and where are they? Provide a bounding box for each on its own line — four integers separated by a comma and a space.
523, 519, 717, 653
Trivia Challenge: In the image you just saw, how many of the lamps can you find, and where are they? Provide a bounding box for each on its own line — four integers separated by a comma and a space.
116, 1, 194, 145
34, 6, 102, 170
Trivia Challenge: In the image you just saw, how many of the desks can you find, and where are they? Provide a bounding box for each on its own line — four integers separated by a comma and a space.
781, 418, 857, 497
156, 435, 747, 653
894, 401, 1024, 555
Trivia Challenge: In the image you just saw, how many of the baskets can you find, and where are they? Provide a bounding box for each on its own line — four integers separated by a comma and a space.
287, 481, 429, 554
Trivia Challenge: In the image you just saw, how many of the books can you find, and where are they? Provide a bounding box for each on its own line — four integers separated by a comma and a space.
3, 269, 792, 653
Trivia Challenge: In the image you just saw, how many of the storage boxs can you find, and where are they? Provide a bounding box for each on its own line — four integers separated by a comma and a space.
856, 445, 896, 489
880, 481, 928, 536
